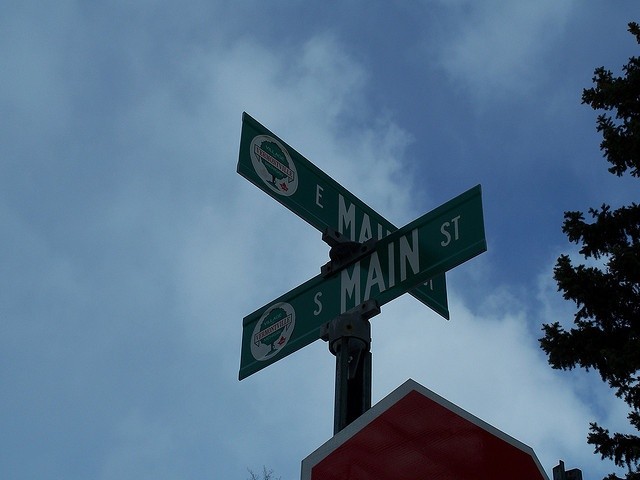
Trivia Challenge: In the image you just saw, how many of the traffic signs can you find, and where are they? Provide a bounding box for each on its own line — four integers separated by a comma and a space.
239, 184, 487, 381
237, 111, 449, 320
